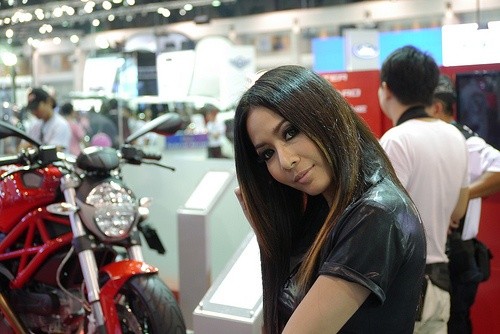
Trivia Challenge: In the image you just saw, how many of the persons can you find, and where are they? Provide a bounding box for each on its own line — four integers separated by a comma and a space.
14, 87, 229, 161
377, 43, 500, 334
234, 66, 427, 334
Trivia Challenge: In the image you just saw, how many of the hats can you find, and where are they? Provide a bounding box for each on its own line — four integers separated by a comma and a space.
433, 75, 456, 104
26, 89, 47, 110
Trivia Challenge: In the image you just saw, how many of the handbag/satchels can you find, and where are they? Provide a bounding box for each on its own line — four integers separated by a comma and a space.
472, 239, 493, 281
427, 263, 451, 290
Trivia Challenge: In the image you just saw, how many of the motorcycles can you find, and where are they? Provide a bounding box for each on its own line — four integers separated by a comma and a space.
0, 112, 191, 334
